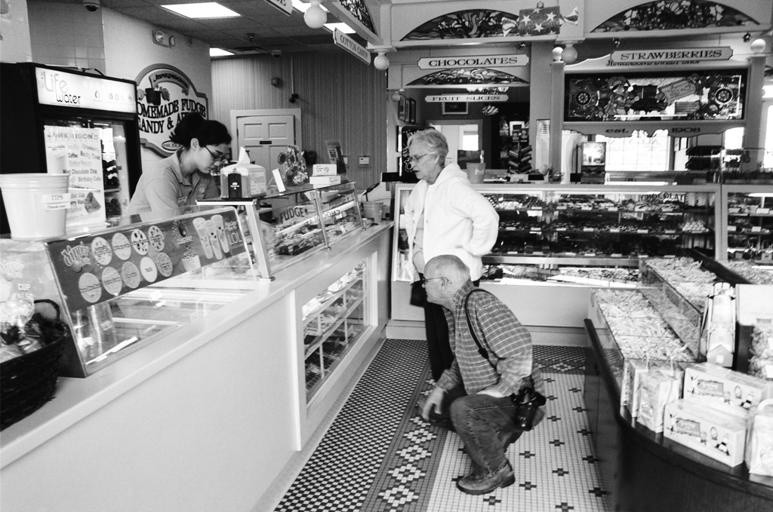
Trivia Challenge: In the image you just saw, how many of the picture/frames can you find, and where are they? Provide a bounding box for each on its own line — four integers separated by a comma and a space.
398, 94, 416, 124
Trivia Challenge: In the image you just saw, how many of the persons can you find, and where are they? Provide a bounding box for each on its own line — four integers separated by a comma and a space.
415, 253, 547, 496
122, 111, 232, 293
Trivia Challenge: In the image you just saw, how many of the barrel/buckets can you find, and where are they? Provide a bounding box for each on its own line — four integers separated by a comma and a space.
0, 174, 69, 242
466, 162, 486, 184
361, 201, 383, 225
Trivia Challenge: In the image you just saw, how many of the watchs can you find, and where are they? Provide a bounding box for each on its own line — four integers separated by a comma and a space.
402, 127, 500, 383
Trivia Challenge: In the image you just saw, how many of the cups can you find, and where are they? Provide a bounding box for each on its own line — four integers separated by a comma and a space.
466, 163, 486, 184
193, 215, 230, 260
363, 200, 388, 225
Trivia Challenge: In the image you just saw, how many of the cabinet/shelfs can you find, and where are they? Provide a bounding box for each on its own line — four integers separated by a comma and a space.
0, 182, 773, 512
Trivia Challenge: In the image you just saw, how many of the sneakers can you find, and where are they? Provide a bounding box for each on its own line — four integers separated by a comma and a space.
456, 459, 515, 495
497, 430, 523, 453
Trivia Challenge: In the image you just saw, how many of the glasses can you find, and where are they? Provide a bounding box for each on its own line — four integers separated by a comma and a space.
204, 145, 221, 161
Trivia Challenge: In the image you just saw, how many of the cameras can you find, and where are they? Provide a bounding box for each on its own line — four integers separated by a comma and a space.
509, 387, 546, 431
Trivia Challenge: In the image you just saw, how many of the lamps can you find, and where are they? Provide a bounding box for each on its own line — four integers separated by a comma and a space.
751, 38, 765, 53
303, 0, 328, 28
152, 30, 176, 47
374, 50, 390, 71
552, 47, 564, 63
562, 42, 578, 63
